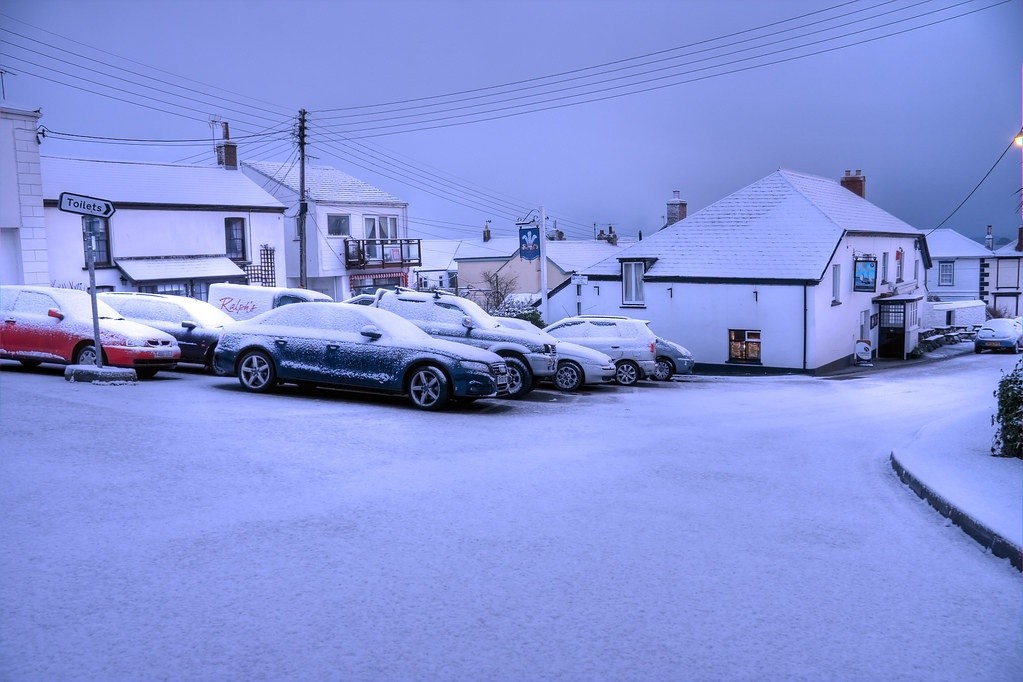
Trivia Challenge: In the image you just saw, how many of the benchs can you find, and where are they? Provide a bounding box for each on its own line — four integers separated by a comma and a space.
922, 335, 944, 341
959, 332, 975, 334
945, 333, 959, 336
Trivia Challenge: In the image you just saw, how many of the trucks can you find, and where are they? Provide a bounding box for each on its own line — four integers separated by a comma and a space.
209, 282, 332, 320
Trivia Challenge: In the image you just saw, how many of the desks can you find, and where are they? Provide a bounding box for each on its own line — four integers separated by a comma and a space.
972, 324, 982, 331
931, 326, 957, 345
919, 329, 935, 351
951, 325, 972, 342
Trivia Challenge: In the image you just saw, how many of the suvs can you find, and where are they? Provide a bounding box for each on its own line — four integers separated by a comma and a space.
95, 292, 237, 369
541, 315, 696, 386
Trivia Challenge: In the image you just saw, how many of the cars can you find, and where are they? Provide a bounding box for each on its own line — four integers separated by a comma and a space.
0, 286, 182, 379
974, 317, 1023, 354
340, 284, 617, 410
213, 302, 509, 409
